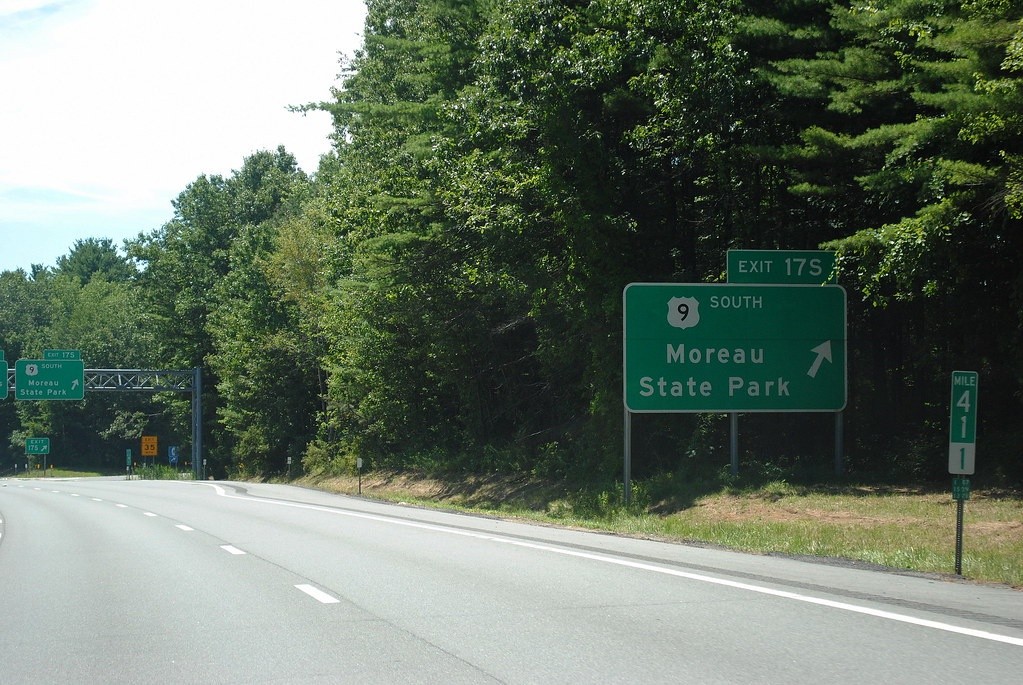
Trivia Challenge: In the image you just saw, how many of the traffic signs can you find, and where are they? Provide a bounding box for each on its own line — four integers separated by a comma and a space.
15, 360, 84, 400
622, 283, 848, 413
